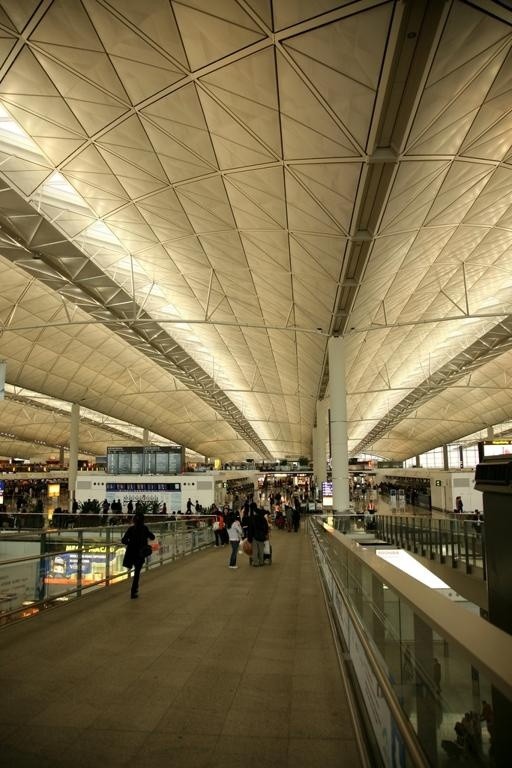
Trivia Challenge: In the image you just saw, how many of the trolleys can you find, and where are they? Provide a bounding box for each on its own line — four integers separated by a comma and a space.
247, 531, 274, 567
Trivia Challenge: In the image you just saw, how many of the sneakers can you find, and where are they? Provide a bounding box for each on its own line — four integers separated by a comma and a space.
128, 589, 140, 599
215, 544, 226, 548
228, 565, 238, 569
253, 563, 265, 567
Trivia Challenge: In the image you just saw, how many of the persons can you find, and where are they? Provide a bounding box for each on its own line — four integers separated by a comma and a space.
353, 481, 484, 539
